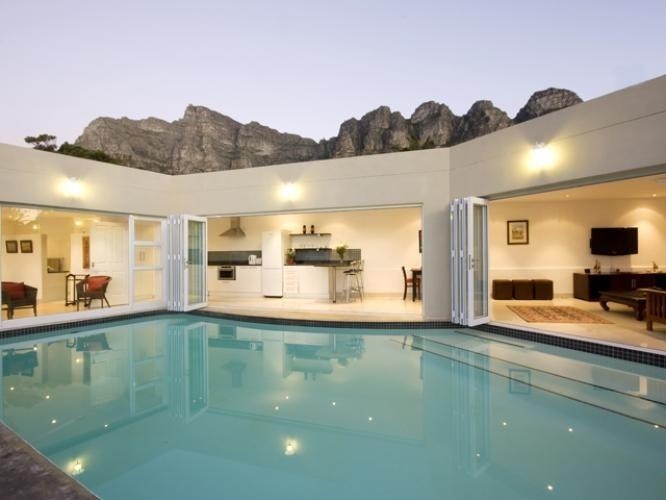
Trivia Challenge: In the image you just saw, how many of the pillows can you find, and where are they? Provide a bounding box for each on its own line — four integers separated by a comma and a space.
1, 281, 24, 299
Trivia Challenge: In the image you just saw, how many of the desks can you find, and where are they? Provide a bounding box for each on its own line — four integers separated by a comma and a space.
412, 269, 424, 299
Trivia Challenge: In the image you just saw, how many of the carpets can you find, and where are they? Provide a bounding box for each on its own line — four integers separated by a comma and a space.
507, 304, 616, 324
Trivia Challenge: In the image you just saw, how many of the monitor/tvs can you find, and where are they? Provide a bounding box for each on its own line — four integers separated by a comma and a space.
590, 227, 638, 256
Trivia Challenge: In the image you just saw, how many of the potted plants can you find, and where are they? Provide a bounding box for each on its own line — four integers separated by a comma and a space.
285, 247, 296, 265
336, 245, 345, 265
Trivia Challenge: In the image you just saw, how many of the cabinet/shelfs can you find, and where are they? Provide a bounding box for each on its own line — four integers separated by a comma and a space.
573, 273, 666, 301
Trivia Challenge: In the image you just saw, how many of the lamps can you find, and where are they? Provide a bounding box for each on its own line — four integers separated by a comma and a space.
8, 207, 40, 226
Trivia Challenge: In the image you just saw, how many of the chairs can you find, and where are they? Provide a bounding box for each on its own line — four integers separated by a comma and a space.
343, 259, 365, 305
76, 333, 112, 351
76, 275, 111, 308
0, 284, 37, 319
637, 286, 666, 329
402, 267, 420, 300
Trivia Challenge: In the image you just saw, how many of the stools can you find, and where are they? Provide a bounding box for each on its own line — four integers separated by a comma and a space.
492, 279, 512, 299
512, 280, 533, 300
533, 278, 553, 300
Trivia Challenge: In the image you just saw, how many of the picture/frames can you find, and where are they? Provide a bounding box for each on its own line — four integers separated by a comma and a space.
6, 240, 18, 253
507, 220, 529, 245
20, 240, 33, 253
419, 229, 423, 253
